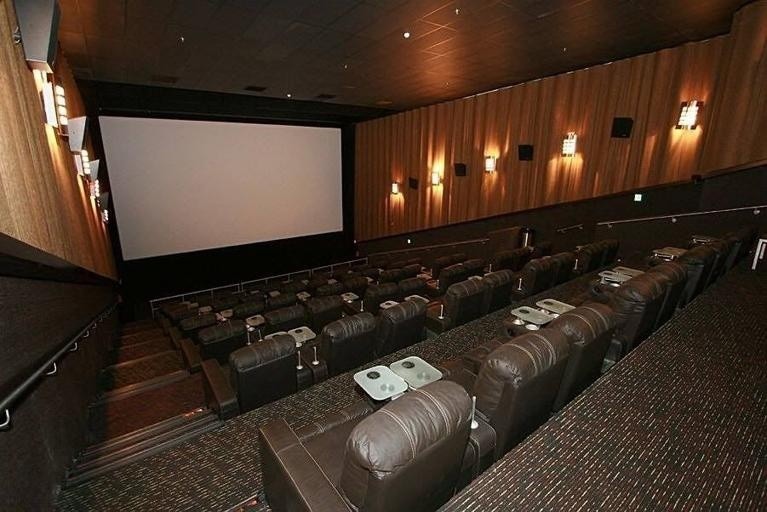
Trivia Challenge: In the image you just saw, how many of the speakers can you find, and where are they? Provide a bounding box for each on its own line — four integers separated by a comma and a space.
610, 117, 633, 138
89, 159, 100, 182
67, 116, 90, 153
100, 192, 109, 209
455, 163, 466, 176
518, 144, 534, 160
14, 0, 61, 74
409, 177, 418, 189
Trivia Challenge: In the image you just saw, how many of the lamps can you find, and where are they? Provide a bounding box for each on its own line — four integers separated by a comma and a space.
67, 115, 110, 211
11, 1, 67, 72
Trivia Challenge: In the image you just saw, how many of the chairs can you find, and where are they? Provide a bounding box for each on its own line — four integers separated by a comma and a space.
153, 218, 618, 418
391, 326, 570, 465
580, 219, 758, 364
256, 365, 480, 511
